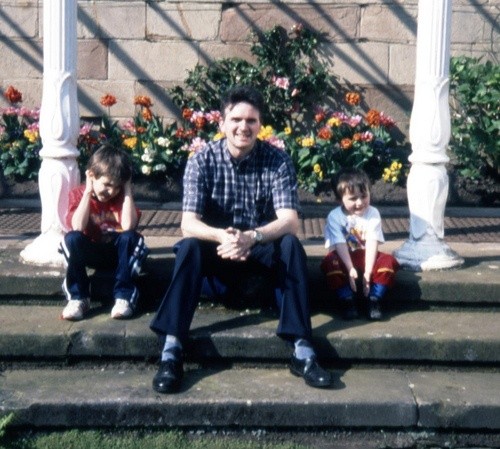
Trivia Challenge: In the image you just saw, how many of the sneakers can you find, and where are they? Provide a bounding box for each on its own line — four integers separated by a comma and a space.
111, 298, 132, 318
61, 298, 92, 320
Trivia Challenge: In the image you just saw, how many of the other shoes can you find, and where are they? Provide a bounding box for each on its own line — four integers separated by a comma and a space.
341, 302, 359, 320
366, 297, 381, 321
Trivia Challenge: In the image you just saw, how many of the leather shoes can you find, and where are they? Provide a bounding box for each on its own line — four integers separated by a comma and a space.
152, 359, 181, 393
288, 354, 332, 387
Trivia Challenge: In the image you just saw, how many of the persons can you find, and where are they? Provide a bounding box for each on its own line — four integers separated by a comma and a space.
57, 144, 150, 321
150, 87, 332, 393
320, 168, 400, 320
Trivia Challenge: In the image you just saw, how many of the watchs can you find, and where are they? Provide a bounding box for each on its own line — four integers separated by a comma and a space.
251, 228, 263, 245
